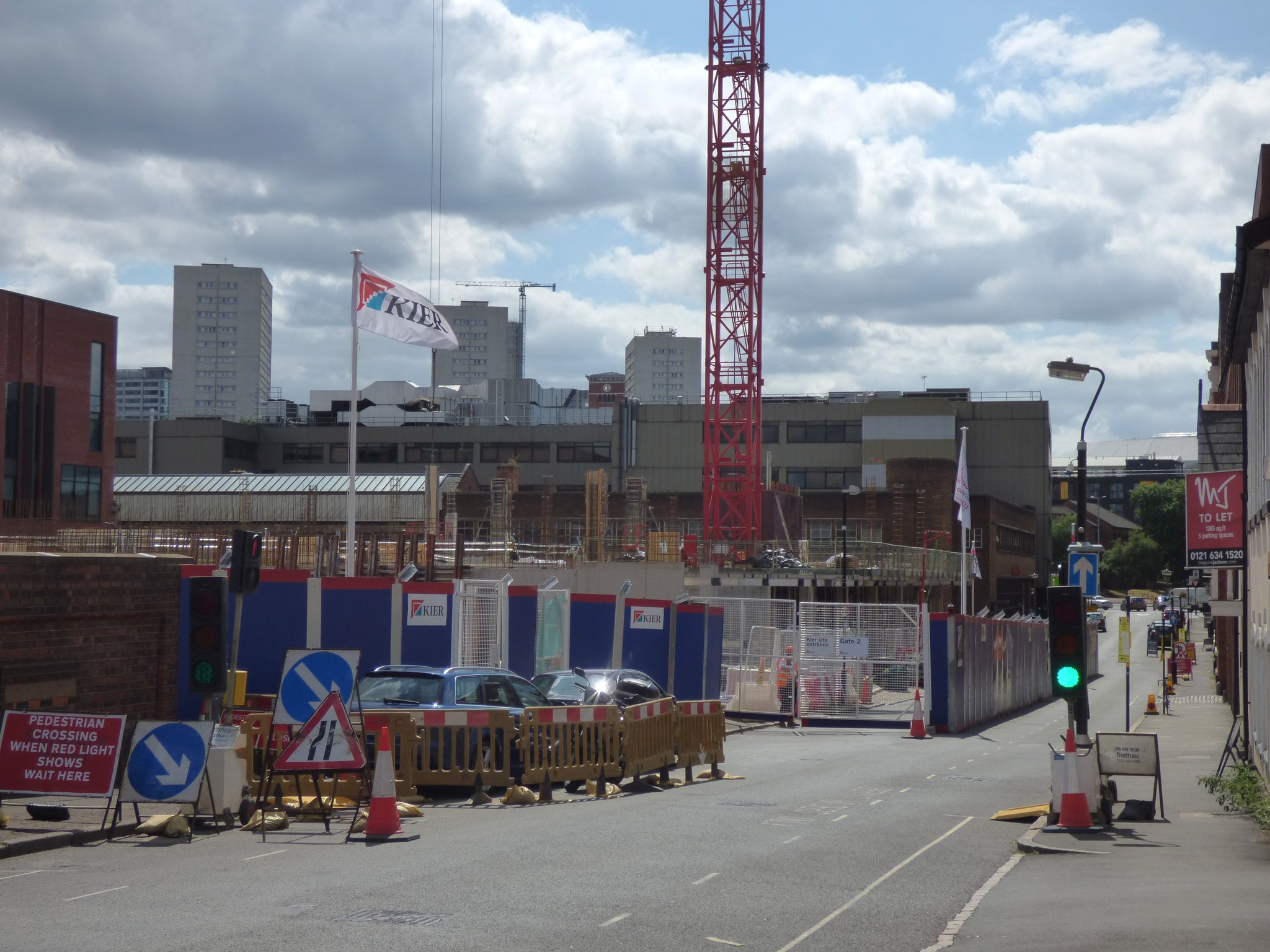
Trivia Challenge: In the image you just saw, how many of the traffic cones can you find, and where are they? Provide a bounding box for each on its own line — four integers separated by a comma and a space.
859, 679, 870, 710
900, 688, 934, 741
1143, 694, 1159, 715
1165, 674, 1176, 695
346, 726, 420, 844
1042, 726, 1105, 834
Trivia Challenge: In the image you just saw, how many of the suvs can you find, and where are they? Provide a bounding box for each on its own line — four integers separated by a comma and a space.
1152, 595, 1166, 611
350, 661, 568, 799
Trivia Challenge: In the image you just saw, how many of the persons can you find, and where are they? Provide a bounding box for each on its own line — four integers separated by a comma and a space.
512, 546, 519, 561
776, 646, 801, 728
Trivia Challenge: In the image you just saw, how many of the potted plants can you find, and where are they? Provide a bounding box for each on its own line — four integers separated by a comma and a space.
563, 550, 576, 569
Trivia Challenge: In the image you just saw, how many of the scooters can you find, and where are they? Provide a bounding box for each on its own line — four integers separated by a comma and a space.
561, 665, 659, 794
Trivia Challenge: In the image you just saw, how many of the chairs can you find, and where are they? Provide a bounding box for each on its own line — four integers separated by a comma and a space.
1136, 598, 1141, 601
1175, 611, 1179, 615
474, 684, 506, 705
1156, 627, 1163, 632
1168, 627, 1173, 633
1167, 612, 1171, 615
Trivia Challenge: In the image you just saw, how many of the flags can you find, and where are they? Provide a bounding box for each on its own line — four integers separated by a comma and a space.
953, 440, 972, 531
357, 262, 459, 351
971, 543, 982, 580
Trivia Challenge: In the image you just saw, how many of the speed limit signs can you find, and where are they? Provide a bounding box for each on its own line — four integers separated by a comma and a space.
839, 606, 851, 617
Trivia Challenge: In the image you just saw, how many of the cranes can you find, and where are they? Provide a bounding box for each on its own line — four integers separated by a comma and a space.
454, 279, 560, 379
703, 1, 771, 566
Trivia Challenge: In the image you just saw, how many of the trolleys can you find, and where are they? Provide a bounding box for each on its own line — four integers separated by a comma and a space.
178, 747, 258, 829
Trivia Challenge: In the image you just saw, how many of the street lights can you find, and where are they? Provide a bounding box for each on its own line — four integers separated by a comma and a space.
1090, 494, 1108, 573
1045, 354, 1109, 749
841, 484, 863, 603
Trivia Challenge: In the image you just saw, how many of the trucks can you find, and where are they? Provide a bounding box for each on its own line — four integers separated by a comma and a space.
1157, 587, 1210, 613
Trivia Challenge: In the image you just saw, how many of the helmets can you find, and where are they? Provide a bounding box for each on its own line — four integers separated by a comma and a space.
785, 646, 793, 654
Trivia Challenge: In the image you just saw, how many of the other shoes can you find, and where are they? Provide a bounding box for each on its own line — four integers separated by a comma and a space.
779, 721, 786, 727
787, 721, 800, 726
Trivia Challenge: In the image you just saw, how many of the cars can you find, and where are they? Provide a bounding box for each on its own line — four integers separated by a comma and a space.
1146, 606, 1188, 647
1086, 611, 1107, 633
1120, 596, 1147, 612
526, 668, 677, 761
1086, 596, 1114, 610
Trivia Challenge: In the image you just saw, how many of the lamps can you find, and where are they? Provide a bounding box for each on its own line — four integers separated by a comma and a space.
1019, 615, 1026, 619
1043, 618, 1049, 625
1033, 615, 1042, 623
496, 572, 514, 590
673, 591, 689, 604
620, 578, 632, 597
992, 609, 1006, 620
220, 549, 232, 569
398, 561, 418, 582
978, 605, 989, 617
1010, 612, 1020, 621
1023, 614, 1032, 623
538, 573, 559, 591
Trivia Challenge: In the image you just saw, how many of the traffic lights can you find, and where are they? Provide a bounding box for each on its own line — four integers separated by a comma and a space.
1046, 584, 1088, 699
226, 529, 264, 593
186, 575, 229, 694
